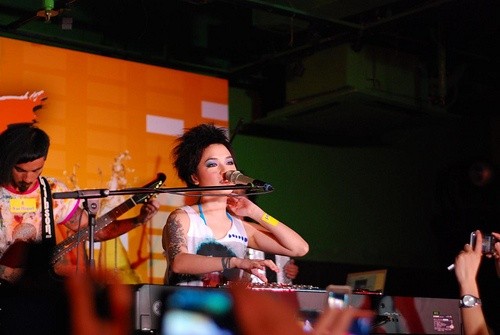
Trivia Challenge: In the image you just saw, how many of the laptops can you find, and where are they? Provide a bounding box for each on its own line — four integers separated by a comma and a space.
345, 267, 390, 293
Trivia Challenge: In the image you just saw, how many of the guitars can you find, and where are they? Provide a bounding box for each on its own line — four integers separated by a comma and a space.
55, 172, 167, 268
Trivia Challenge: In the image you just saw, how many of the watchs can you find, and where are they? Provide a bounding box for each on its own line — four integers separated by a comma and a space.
458, 294, 481, 308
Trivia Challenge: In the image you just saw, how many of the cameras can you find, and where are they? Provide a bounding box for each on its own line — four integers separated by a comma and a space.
470, 232, 496, 254
153, 288, 242, 335
348, 316, 372, 335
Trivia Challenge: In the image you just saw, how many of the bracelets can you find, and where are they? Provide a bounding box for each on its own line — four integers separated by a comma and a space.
221, 256, 232, 271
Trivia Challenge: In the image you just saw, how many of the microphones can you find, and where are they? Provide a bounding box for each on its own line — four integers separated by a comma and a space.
223, 170, 272, 191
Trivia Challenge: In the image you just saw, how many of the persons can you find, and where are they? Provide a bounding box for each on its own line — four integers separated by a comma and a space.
227, 189, 297, 282
0, 125, 159, 283
310, 306, 356, 335
162, 124, 309, 286
225, 185, 298, 284
454, 230, 500, 335
